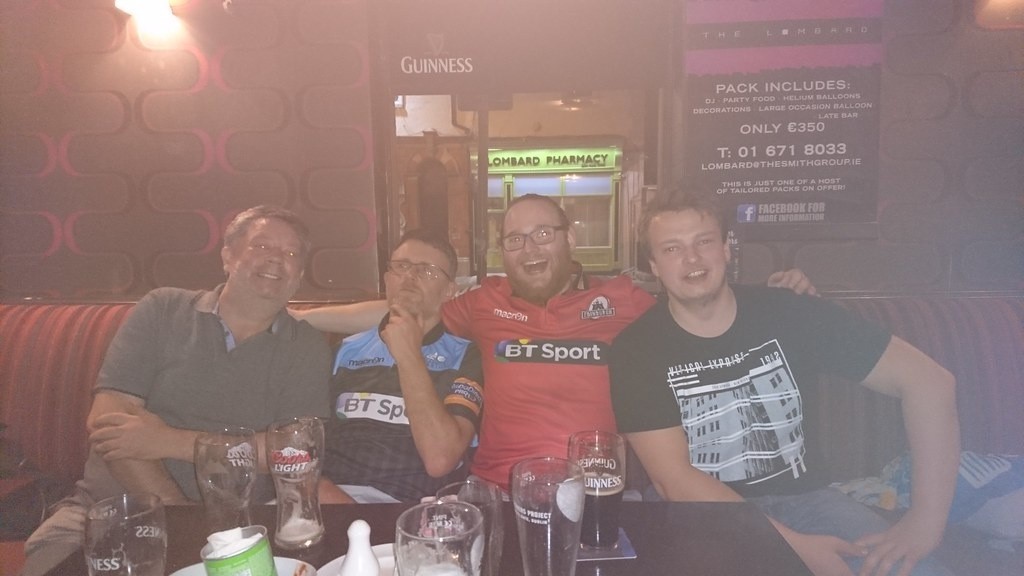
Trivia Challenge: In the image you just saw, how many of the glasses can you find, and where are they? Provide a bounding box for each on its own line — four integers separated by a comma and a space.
496, 225, 564, 251
386, 260, 452, 281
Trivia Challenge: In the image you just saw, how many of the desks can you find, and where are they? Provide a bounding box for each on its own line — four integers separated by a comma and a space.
0, 483, 809, 576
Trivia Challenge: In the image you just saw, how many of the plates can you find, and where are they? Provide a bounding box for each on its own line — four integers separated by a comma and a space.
318, 543, 467, 576
170, 557, 316, 576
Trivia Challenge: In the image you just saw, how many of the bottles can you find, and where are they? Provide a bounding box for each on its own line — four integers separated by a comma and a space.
419, 130, 450, 243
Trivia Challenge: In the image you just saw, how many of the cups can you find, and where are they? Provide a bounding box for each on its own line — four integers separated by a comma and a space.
200, 525, 277, 576
570, 431, 626, 551
513, 457, 584, 576
85, 492, 166, 576
265, 417, 325, 550
195, 426, 258, 532
438, 481, 504, 576
396, 501, 485, 576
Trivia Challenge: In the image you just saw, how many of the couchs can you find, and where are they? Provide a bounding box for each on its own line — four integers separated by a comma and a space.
0, 303, 331, 500
781, 293, 1024, 541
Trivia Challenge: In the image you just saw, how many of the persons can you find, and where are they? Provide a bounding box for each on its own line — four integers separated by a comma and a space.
286, 194, 817, 506
610, 191, 961, 576
21, 205, 331, 576
